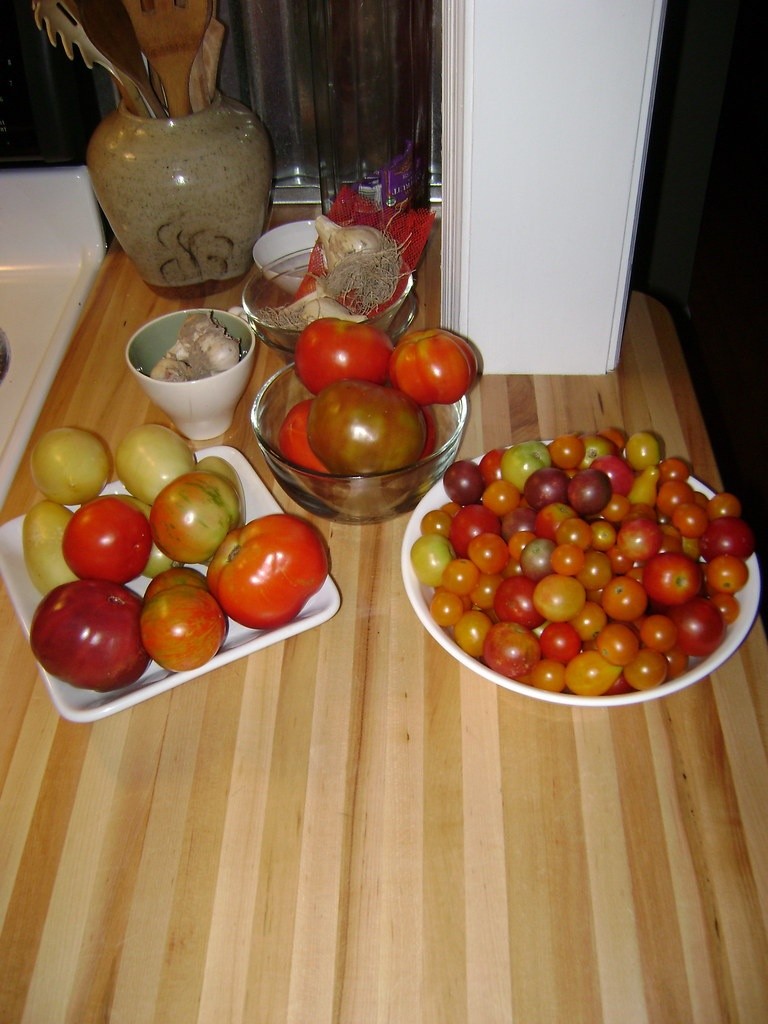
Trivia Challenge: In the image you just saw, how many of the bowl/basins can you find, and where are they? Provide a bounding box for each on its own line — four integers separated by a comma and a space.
249, 361, 468, 526
242, 219, 420, 365
125, 308, 256, 441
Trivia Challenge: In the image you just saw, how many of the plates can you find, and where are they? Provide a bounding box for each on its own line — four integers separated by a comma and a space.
0, 446, 341, 723
400, 438, 762, 708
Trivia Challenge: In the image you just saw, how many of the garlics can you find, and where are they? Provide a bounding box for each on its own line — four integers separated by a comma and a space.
258, 214, 393, 326
147, 312, 239, 384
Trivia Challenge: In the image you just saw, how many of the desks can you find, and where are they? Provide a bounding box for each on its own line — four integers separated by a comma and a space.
0, 199, 767, 1024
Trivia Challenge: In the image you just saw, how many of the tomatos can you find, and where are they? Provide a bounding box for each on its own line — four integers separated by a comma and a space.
279, 317, 479, 514
22, 422, 329, 692
409, 426, 754, 698
